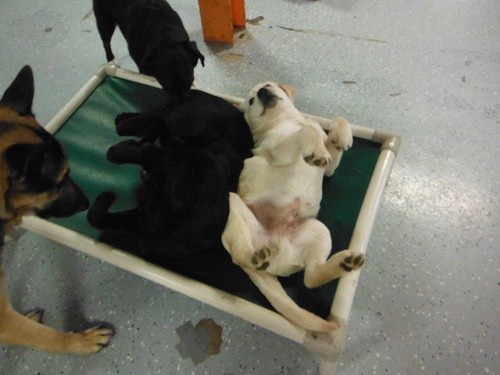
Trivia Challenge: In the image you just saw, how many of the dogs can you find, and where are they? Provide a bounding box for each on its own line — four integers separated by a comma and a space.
92, 0, 205, 90
0, 65, 116, 355
86, 81, 364, 331
